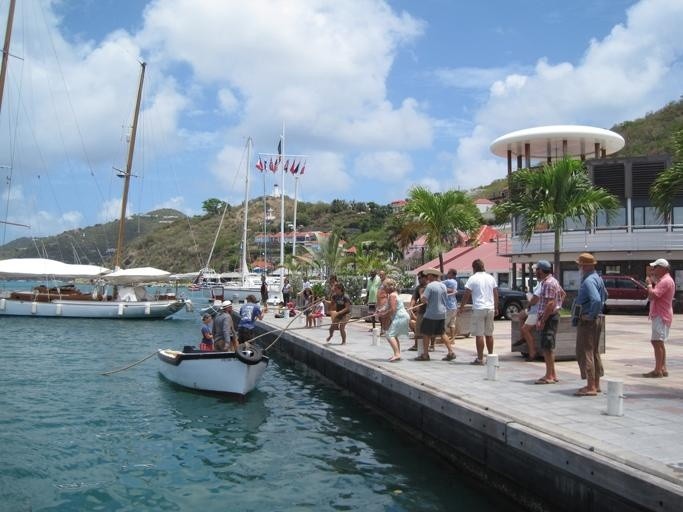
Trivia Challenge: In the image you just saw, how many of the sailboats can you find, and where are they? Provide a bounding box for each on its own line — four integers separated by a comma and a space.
197, 117, 309, 304
0, 1, 191, 322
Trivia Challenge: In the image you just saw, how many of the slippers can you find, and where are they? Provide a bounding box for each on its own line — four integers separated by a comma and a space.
574, 387, 601, 396
535, 379, 559, 384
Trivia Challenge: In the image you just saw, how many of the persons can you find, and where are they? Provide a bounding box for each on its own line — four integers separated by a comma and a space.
520, 268, 543, 362
571, 252, 608, 396
534, 260, 566, 384
643, 258, 676, 378
200, 269, 459, 361
457, 260, 499, 366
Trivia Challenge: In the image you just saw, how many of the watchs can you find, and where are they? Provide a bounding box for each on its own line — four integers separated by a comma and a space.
540, 318, 546, 324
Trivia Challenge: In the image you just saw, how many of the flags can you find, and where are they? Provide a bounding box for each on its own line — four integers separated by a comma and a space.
256, 138, 306, 176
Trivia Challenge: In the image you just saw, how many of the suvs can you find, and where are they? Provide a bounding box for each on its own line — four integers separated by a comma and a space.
436, 272, 530, 320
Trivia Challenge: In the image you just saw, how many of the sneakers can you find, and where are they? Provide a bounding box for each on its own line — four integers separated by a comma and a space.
642, 371, 668, 378
470, 358, 484, 365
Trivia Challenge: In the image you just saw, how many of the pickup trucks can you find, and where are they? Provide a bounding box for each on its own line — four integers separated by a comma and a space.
561, 272, 676, 315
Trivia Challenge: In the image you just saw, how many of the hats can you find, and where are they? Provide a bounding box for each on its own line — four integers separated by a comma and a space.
649, 258, 668, 267
537, 260, 552, 271
423, 267, 443, 276
575, 253, 597, 265
220, 300, 232, 310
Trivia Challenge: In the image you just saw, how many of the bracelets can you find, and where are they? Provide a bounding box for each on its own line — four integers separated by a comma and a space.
651, 282, 656, 288
646, 284, 653, 288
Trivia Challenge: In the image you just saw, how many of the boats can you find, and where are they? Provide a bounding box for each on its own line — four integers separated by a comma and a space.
187, 285, 200, 291
156, 339, 270, 405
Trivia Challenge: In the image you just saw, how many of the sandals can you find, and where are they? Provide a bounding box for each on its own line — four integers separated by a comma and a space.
442, 352, 456, 360
414, 354, 430, 361
